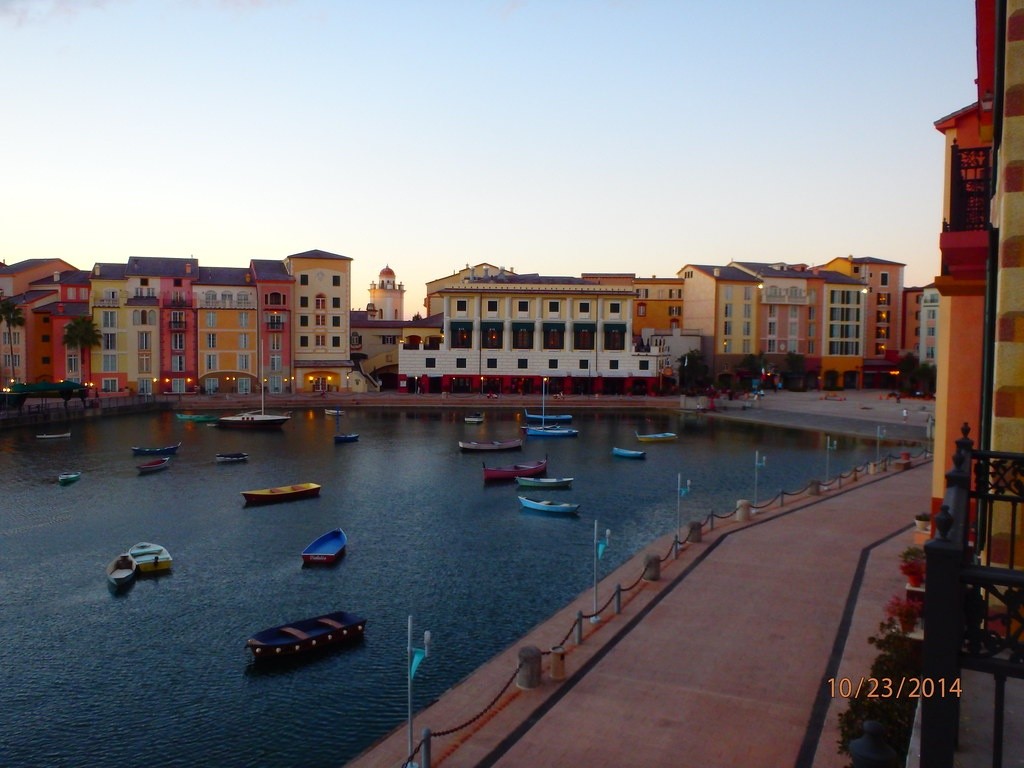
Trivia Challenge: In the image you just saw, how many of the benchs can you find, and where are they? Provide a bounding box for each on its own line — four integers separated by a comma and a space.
517, 464, 532, 468
271, 489, 284, 493
292, 485, 305, 490
561, 503, 569, 506
540, 500, 551, 505
318, 618, 344, 629
471, 442, 477, 444
131, 548, 169, 562
493, 441, 499, 444
280, 627, 310, 640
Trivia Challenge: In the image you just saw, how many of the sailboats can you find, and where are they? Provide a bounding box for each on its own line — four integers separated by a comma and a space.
521, 377, 580, 436
216, 339, 291, 428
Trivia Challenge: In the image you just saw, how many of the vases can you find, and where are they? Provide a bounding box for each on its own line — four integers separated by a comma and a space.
901, 623, 913, 633
909, 576, 922, 587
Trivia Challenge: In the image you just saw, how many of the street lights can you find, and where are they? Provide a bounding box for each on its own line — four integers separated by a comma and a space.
589, 520, 611, 624
826, 435, 838, 492
404, 615, 432, 768
753, 450, 766, 514
876, 425, 886, 473
677, 472, 691, 552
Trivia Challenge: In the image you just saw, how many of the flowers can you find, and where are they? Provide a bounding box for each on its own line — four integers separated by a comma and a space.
900, 559, 925, 576
885, 596, 923, 625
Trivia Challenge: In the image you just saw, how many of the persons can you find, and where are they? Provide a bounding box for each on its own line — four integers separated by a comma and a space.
778, 380, 783, 391
696, 402, 701, 412
902, 408, 908, 422
744, 388, 765, 400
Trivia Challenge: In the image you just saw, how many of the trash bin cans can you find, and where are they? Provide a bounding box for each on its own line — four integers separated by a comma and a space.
549, 647, 567, 680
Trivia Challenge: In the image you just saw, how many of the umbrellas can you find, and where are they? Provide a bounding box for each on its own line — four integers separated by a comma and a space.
12, 380, 88, 411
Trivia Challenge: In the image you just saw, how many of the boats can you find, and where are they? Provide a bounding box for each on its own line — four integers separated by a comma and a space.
243, 611, 367, 666
518, 495, 581, 514
58, 471, 81, 484
302, 528, 347, 567
131, 441, 182, 455
137, 457, 170, 473
176, 413, 219, 423
634, 430, 679, 442
458, 438, 523, 453
514, 477, 574, 488
482, 453, 548, 481
523, 409, 573, 424
324, 409, 346, 416
128, 542, 173, 573
612, 447, 647, 458
215, 452, 249, 463
334, 433, 360, 442
106, 552, 137, 587
464, 411, 485, 424
240, 482, 322, 506
36, 432, 71, 439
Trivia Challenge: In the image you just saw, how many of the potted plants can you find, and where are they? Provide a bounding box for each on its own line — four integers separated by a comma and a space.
914, 512, 930, 529
